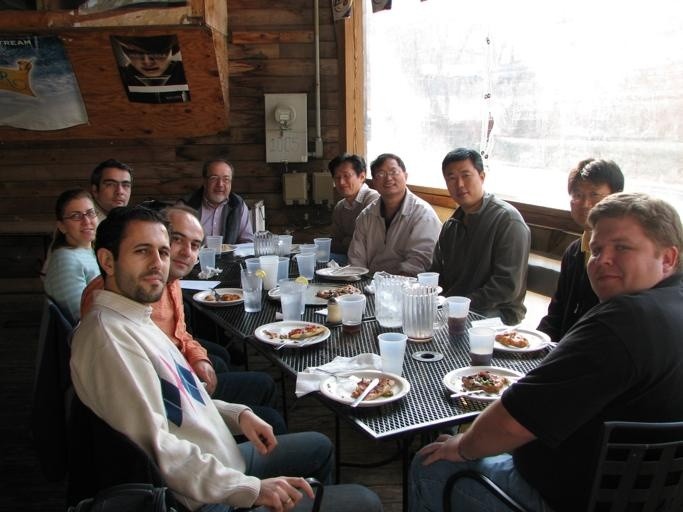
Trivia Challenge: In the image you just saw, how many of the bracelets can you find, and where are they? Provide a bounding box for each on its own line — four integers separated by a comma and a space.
457, 436, 480, 462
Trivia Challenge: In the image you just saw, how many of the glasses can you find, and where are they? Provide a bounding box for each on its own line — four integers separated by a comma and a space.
64, 209, 100, 222
375, 169, 402, 178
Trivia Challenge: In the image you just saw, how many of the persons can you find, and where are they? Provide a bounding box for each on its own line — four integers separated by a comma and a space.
42, 188, 231, 374
403, 190, 682, 512
68, 203, 385, 512
326, 155, 382, 255
117, 35, 185, 108
345, 153, 444, 276
536, 158, 623, 343
81, 200, 277, 407
47, 157, 136, 256
424, 147, 529, 440
182, 159, 255, 367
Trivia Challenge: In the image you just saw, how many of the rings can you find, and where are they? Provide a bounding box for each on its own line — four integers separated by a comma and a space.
283, 497, 291, 504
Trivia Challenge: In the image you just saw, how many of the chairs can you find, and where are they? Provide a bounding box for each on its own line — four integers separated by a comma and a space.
66, 328, 323, 512
441, 420, 683, 511
35, 297, 212, 411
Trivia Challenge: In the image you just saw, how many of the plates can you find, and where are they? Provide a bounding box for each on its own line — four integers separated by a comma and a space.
491, 325, 550, 352
318, 370, 409, 407
194, 287, 243, 307
441, 365, 526, 402
253, 320, 331, 349
315, 265, 369, 280
265, 280, 362, 309
223, 244, 239, 253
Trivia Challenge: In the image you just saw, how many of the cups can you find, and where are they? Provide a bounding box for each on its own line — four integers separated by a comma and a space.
276, 277, 306, 321
244, 255, 289, 289
252, 230, 276, 255
445, 295, 472, 334
199, 233, 223, 278
279, 234, 293, 253
296, 235, 332, 279
372, 269, 412, 330
466, 327, 496, 364
334, 293, 367, 334
398, 286, 445, 341
417, 272, 439, 286
375, 332, 410, 376
240, 268, 263, 314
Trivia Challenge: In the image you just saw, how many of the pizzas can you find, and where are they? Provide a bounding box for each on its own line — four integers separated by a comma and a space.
352, 377, 398, 402
462, 369, 508, 395
496, 331, 529, 349
288, 325, 324, 339
315, 285, 361, 301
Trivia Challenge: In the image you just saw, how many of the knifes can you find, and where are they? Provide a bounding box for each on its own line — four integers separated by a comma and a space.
350, 376, 380, 407
449, 386, 485, 400
